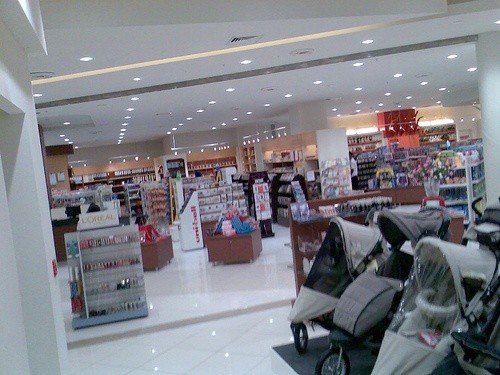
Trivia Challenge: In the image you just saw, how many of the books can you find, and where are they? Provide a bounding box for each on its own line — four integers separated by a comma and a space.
348, 124, 457, 152
69, 145, 351, 222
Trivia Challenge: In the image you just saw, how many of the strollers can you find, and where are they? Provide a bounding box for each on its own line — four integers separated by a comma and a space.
287, 197, 500, 375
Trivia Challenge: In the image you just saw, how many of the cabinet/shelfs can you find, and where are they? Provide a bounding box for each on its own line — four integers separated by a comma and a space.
230, 171, 276, 238
345, 120, 456, 190
204, 225, 265, 267
62, 224, 150, 331
140, 235, 174, 273
111, 182, 144, 227
436, 157, 485, 229
185, 129, 322, 177
284, 190, 392, 311
71, 166, 158, 190
271, 173, 308, 229
